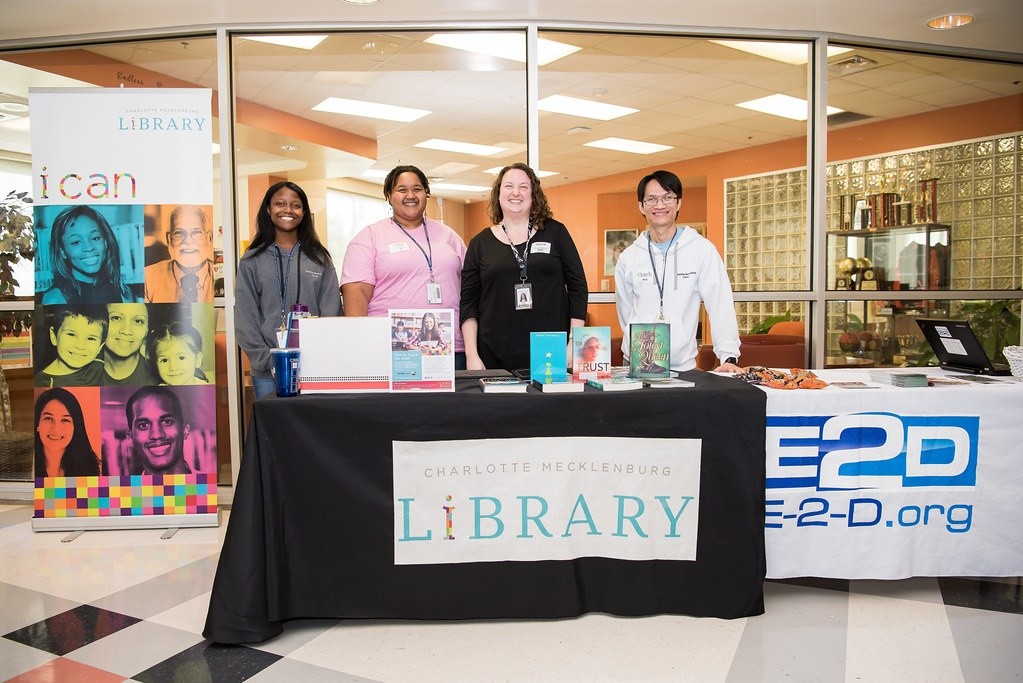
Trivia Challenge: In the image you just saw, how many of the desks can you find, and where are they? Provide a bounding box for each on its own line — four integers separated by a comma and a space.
200, 367, 1023, 647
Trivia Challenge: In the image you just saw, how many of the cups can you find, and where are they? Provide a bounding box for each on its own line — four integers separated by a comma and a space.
269, 347, 300, 397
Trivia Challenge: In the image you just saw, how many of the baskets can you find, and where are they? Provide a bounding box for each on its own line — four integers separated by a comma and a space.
1003, 345, 1023, 381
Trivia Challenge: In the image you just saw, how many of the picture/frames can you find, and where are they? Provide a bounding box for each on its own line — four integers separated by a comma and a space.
604, 228, 638, 277
645, 222, 707, 239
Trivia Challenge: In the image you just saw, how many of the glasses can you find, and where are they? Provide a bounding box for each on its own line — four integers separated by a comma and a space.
169, 229, 208, 242
643, 195, 678, 206
584, 345, 600, 350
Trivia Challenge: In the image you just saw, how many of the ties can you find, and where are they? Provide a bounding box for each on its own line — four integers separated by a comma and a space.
181, 274, 200, 303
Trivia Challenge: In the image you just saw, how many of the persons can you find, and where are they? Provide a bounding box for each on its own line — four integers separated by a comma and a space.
144, 204, 215, 304
126, 386, 204, 475
458, 163, 588, 371
614, 170, 747, 374
34, 387, 101, 478
148, 323, 210, 385
33, 302, 109, 387
100, 303, 207, 386
339, 165, 468, 371
234, 181, 341, 398
392, 312, 450, 354
520, 293, 527, 302
580, 334, 600, 363
42, 206, 135, 305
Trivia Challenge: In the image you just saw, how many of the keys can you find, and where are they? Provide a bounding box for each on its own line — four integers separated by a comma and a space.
280, 325, 285, 340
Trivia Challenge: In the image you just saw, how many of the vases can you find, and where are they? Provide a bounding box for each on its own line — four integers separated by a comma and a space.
838, 332, 860, 356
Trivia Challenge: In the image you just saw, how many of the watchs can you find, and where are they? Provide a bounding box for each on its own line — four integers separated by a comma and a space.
726, 357, 736, 363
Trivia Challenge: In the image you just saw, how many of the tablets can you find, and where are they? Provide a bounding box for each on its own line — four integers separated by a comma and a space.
515, 368, 530, 380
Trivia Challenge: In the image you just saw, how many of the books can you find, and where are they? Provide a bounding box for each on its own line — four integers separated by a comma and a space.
456, 321, 695, 393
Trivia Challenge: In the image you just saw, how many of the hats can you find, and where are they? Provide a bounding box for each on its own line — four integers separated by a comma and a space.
580, 335, 597, 349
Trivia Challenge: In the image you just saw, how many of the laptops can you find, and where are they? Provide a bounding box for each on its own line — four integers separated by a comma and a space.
915, 318, 1013, 376
